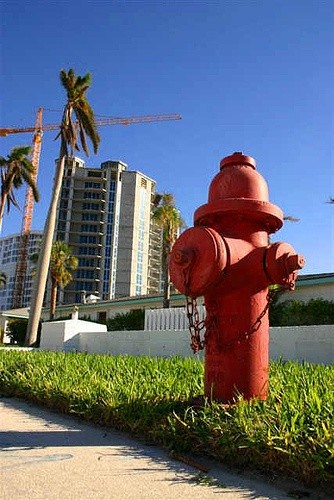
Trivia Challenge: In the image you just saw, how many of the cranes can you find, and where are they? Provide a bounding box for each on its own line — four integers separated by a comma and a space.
0, 108, 182, 310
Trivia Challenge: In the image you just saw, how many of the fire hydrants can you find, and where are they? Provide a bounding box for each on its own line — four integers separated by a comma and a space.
168, 152, 305, 403
